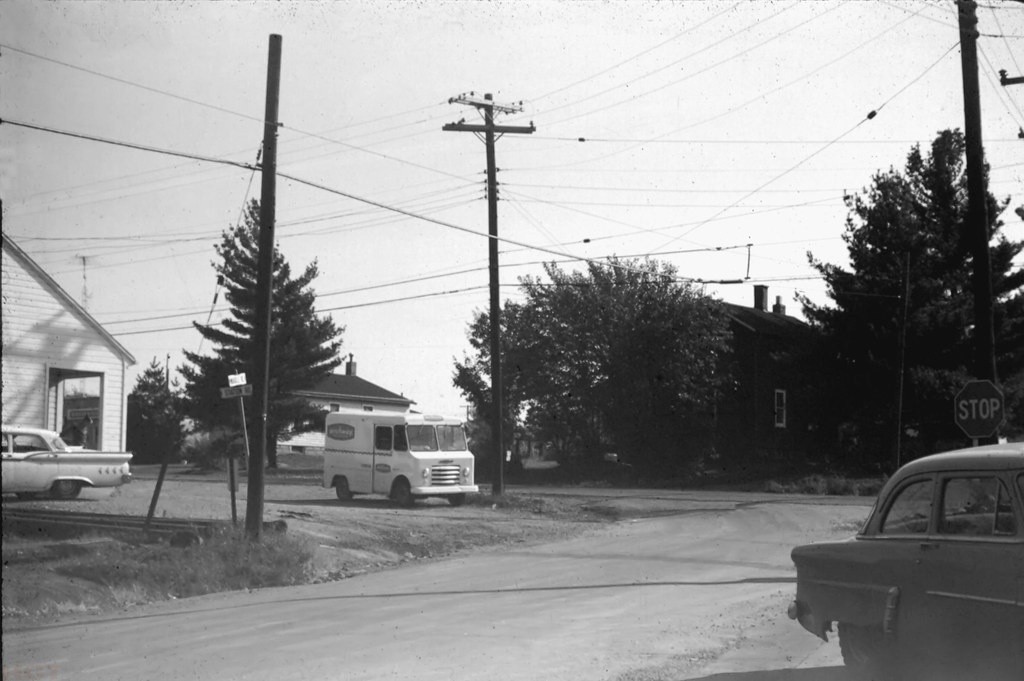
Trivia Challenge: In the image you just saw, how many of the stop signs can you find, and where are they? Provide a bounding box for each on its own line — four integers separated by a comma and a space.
952, 379, 1007, 439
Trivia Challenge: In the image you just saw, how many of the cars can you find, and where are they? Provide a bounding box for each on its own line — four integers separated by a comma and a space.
788, 443, 1024, 680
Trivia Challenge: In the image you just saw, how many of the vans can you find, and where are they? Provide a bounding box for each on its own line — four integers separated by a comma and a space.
325, 411, 480, 508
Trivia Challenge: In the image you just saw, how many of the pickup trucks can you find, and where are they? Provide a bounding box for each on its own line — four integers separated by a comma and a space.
2, 425, 133, 501
